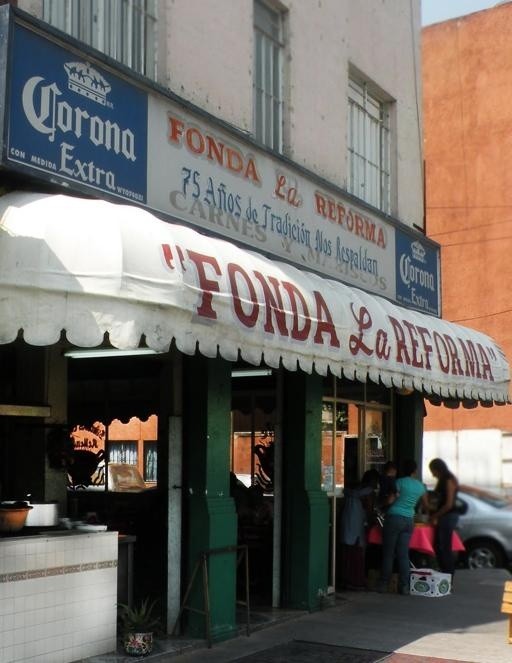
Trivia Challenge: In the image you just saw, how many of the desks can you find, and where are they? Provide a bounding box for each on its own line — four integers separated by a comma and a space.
369, 524, 465, 574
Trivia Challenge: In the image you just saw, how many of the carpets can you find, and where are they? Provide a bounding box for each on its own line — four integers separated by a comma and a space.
229, 637, 472, 662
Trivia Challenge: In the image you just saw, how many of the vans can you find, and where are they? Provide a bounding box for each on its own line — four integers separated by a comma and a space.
75, 464, 146, 494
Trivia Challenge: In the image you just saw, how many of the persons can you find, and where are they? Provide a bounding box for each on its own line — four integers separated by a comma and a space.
340, 459, 459, 595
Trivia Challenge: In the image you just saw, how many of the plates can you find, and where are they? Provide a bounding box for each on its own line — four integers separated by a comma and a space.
78, 523, 108, 533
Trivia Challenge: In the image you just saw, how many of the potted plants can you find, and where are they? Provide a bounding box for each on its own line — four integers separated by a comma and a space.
116, 597, 165, 656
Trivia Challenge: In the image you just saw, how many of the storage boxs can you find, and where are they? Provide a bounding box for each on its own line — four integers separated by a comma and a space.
409, 572, 452, 598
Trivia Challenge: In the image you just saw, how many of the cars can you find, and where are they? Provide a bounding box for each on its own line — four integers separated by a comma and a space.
417, 484, 512, 569
